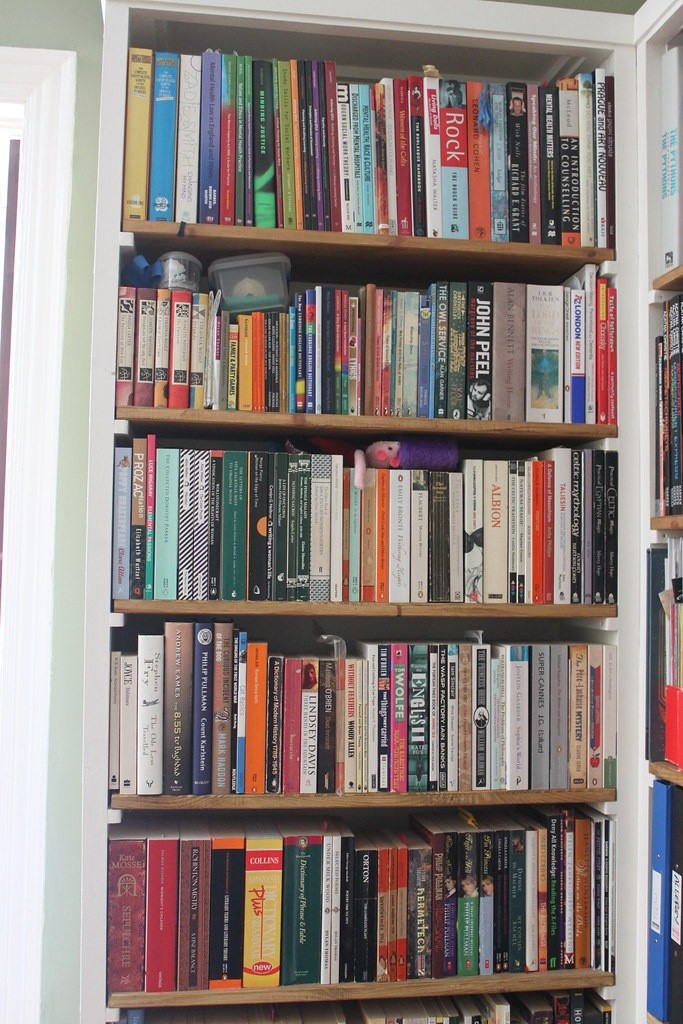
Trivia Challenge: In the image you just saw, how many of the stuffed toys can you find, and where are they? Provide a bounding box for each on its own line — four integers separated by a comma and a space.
285, 438, 401, 489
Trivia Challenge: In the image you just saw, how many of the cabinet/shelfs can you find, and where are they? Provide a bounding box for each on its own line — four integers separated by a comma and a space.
41, 0, 683, 1023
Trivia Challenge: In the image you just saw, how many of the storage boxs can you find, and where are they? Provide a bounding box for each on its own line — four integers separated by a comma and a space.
207, 252, 292, 318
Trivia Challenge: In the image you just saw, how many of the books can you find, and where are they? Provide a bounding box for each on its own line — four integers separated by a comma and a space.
655, 294, 683, 517
659, 46, 683, 277
108, 48, 619, 1024
644, 537, 683, 770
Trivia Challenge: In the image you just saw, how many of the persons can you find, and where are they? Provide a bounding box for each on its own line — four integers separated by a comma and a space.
510, 97, 524, 116
411, 86, 421, 99
445, 875, 457, 898
461, 875, 479, 897
481, 875, 493, 896
467, 379, 491, 419
303, 662, 318, 689
